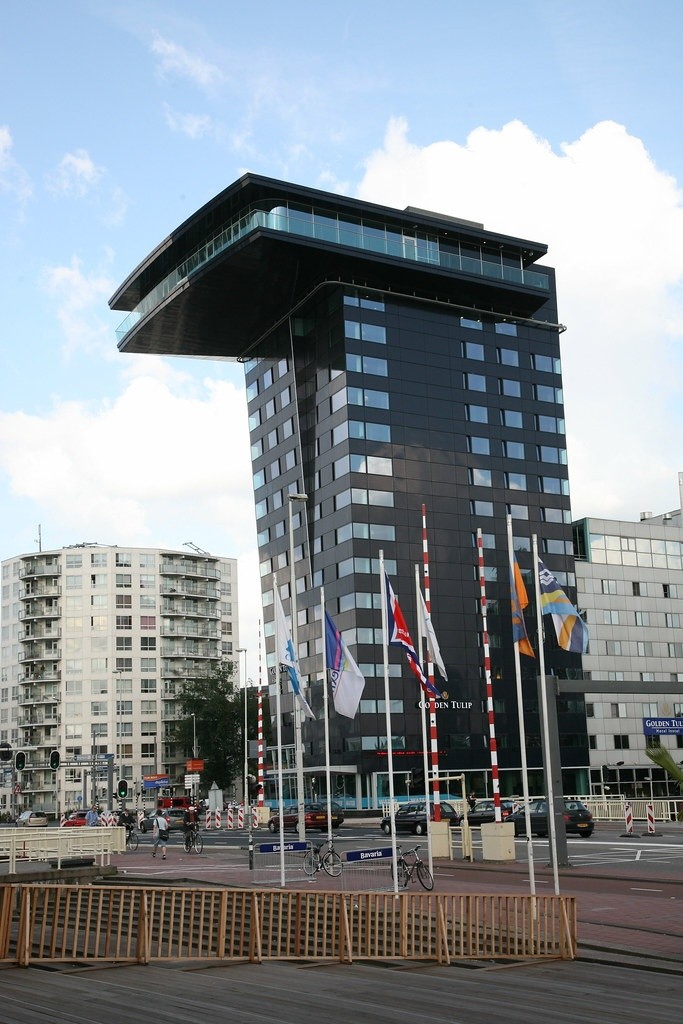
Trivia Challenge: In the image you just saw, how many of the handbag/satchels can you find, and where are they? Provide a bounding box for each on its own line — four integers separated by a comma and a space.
159, 829, 169, 842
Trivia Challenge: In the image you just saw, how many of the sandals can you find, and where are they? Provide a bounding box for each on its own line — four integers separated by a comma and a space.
152, 851, 156, 857
162, 855, 166, 859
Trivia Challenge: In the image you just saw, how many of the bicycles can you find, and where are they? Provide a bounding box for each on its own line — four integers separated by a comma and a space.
390, 843, 435, 891
124, 823, 139, 851
302, 833, 344, 877
183, 821, 203, 854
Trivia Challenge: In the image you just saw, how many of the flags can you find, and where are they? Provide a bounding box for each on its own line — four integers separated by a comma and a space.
508, 552, 536, 657
419, 590, 448, 682
274, 588, 316, 720
385, 573, 442, 697
537, 557, 590, 654
325, 611, 365, 720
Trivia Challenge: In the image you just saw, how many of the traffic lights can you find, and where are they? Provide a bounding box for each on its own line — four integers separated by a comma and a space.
336, 774, 345, 790
116, 779, 129, 799
49, 750, 60, 770
250, 783, 262, 796
15, 751, 26, 771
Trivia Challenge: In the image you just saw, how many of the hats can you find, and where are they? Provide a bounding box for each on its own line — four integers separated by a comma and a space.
189, 807, 194, 811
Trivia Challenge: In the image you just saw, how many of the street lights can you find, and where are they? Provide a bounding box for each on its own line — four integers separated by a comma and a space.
112, 669, 123, 812
235, 647, 250, 831
286, 492, 308, 851
191, 713, 197, 807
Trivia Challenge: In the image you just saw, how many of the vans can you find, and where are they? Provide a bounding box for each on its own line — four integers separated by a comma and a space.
156, 796, 202, 815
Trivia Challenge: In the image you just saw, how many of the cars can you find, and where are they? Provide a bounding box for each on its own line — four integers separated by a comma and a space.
380, 800, 460, 837
268, 803, 332, 833
458, 800, 522, 827
14, 810, 49, 827
504, 798, 596, 838
60, 812, 87, 827
100, 810, 124, 826
321, 801, 345, 829
139, 808, 188, 834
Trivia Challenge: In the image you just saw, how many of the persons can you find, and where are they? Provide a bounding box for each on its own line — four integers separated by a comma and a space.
84, 805, 98, 826
468, 791, 476, 809
183, 806, 201, 848
118, 810, 137, 830
152, 810, 168, 860
